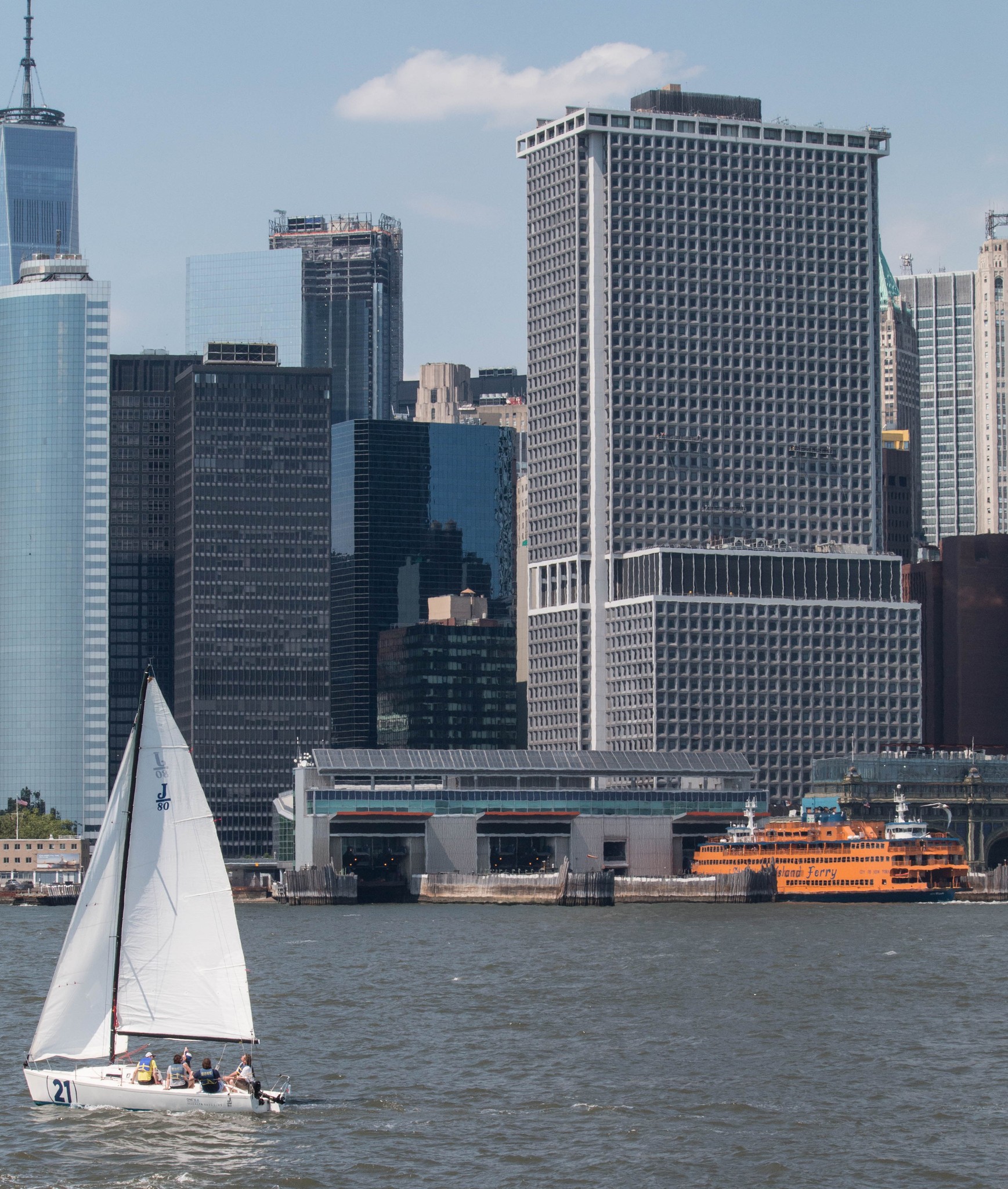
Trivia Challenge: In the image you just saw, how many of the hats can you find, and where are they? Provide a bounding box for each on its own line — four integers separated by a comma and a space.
145, 1052, 156, 1058
186, 1052, 192, 1062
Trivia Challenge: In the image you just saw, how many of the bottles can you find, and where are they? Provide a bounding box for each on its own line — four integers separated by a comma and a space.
288, 1083, 291, 1094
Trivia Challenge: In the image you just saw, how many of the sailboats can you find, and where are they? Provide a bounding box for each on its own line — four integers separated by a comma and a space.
20, 654, 293, 1117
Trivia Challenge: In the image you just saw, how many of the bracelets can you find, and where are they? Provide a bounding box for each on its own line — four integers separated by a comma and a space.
131, 1082, 134, 1083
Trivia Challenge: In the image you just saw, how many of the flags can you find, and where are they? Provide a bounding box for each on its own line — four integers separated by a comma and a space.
17, 796, 28, 805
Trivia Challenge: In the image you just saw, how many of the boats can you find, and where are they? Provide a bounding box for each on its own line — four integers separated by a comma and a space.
689, 732, 975, 903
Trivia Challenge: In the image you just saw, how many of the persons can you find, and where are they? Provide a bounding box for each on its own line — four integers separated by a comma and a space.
129, 1046, 255, 1095
